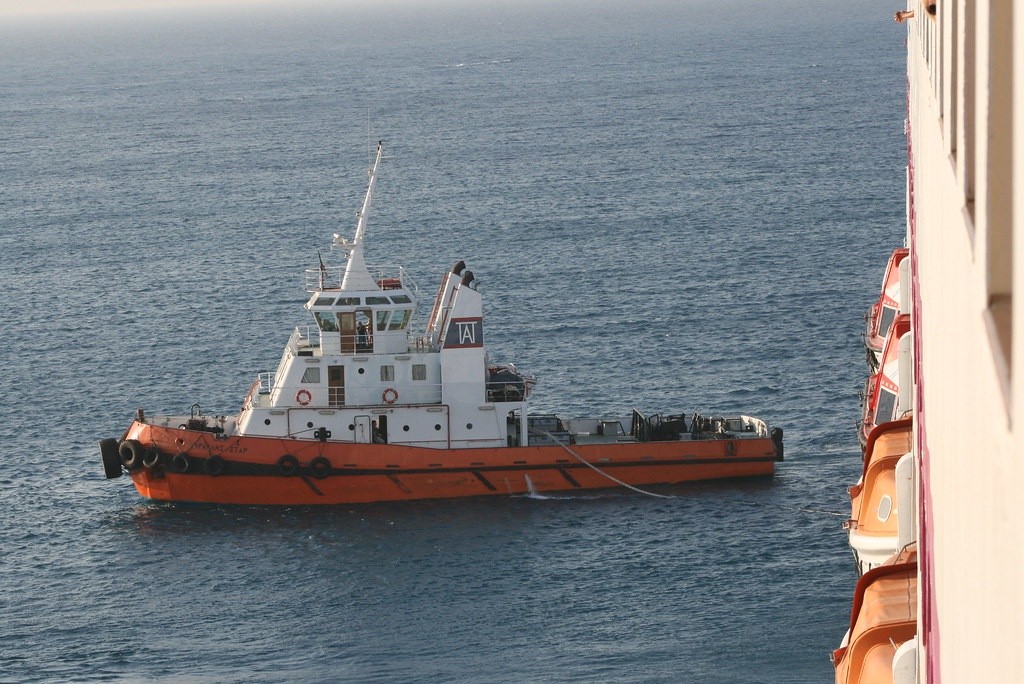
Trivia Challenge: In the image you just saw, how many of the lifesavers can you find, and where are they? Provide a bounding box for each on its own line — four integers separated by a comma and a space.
296, 389, 312, 405
382, 388, 398, 404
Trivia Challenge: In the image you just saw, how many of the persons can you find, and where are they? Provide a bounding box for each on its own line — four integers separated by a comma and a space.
370, 420, 383, 445
357, 322, 369, 348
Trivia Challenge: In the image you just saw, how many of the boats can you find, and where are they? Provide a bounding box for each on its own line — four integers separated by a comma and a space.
828, 247, 917, 684
100, 139, 785, 506
